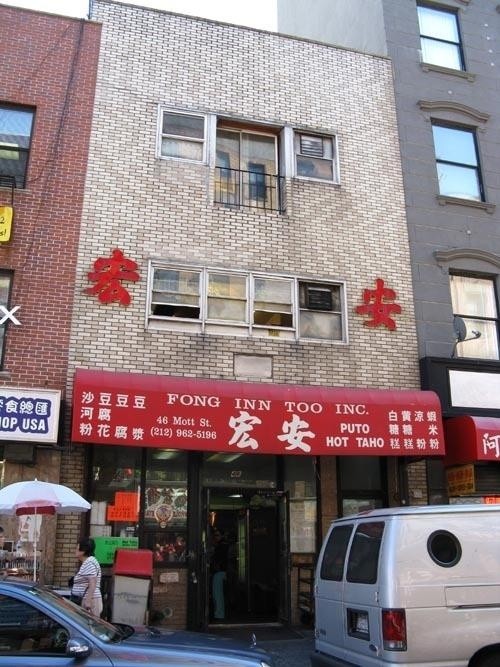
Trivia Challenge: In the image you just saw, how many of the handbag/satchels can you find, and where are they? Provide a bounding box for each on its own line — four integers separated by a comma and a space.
70, 594, 82, 605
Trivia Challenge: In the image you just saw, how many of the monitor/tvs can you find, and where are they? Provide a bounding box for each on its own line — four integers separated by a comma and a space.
2, 541, 14, 553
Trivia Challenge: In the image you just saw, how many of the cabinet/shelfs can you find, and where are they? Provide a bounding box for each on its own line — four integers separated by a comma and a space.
295, 561, 315, 627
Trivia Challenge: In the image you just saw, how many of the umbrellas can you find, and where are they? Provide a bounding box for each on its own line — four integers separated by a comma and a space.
0, 477, 91, 581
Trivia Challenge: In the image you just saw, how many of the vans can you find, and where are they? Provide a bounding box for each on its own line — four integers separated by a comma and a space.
313, 504, 500, 666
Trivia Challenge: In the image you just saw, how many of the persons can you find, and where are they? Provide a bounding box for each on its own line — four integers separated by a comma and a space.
210, 528, 229, 620
70, 538, 104, 618
0, 526, 8, 568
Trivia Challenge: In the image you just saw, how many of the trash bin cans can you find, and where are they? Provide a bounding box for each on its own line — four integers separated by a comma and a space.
110, 548, 153, 626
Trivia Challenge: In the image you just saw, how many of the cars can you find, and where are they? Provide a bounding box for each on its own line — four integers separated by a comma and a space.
0, 577, 273, 666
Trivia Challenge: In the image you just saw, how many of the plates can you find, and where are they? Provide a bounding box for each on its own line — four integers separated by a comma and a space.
155, 504, 173, 523
